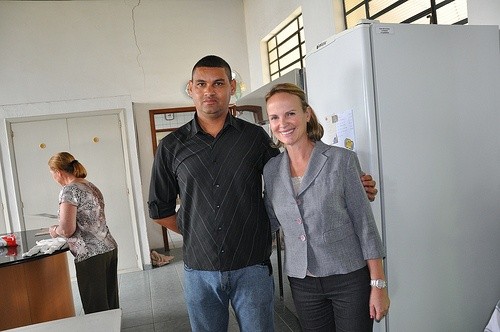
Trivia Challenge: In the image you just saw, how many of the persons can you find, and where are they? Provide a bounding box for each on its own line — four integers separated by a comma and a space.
148, 54, 378, 332
244, 83, 391, 332
47, 149, 120, 314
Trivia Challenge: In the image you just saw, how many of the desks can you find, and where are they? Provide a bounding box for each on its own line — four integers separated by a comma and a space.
0, 228, 85, 331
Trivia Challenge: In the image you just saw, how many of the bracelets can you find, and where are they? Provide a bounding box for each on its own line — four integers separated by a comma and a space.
54, 225, 61, 236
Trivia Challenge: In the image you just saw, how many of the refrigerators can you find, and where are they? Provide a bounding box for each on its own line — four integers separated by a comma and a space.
302, 18, 500, 332
234, 68, 305, 322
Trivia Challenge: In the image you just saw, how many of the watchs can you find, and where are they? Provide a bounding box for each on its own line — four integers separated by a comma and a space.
369, 277, 388, 290
